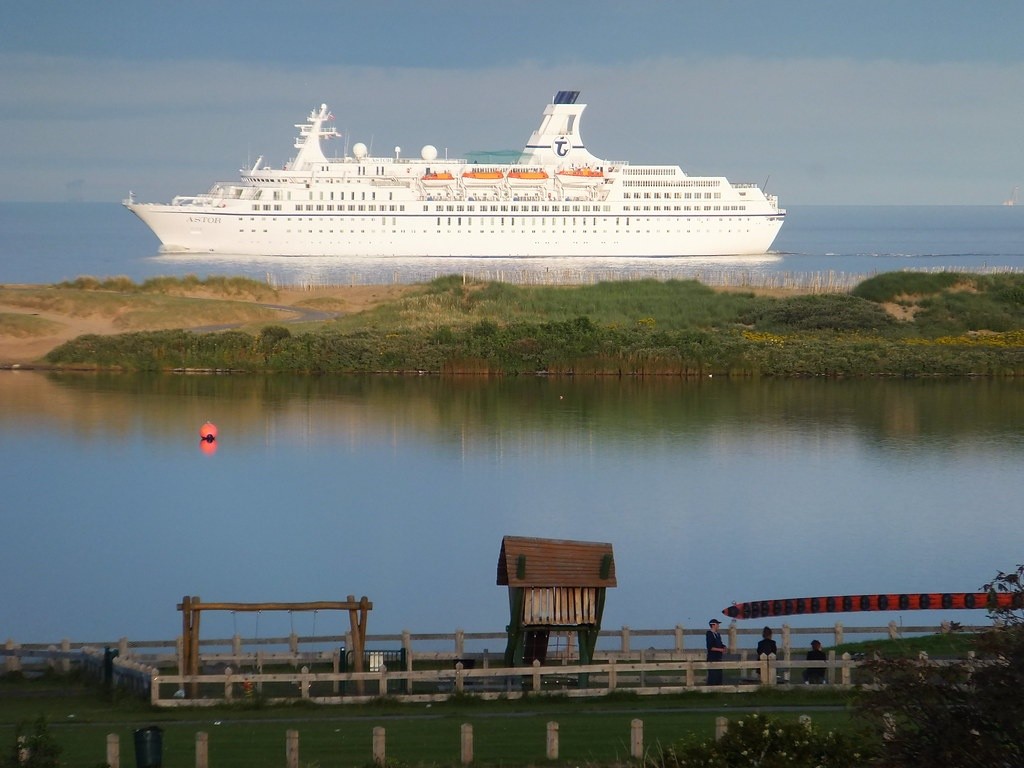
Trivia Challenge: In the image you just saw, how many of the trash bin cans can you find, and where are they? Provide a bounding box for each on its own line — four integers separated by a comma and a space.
132, 725, 164, 768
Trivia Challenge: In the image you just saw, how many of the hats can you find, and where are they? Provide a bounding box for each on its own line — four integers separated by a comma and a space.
709, 619, 722, 627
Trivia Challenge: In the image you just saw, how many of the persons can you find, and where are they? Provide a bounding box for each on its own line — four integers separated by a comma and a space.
756, 627, 781, 679
706, 619, 727, 686
803, 640, 826, 684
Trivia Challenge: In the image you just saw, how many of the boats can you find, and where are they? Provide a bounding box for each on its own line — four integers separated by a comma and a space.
507, 168, 548, 186
420, 173, 455, 187
556, 173, 604, 188
462, 171, 503, 187
121, 85, 786, 257
